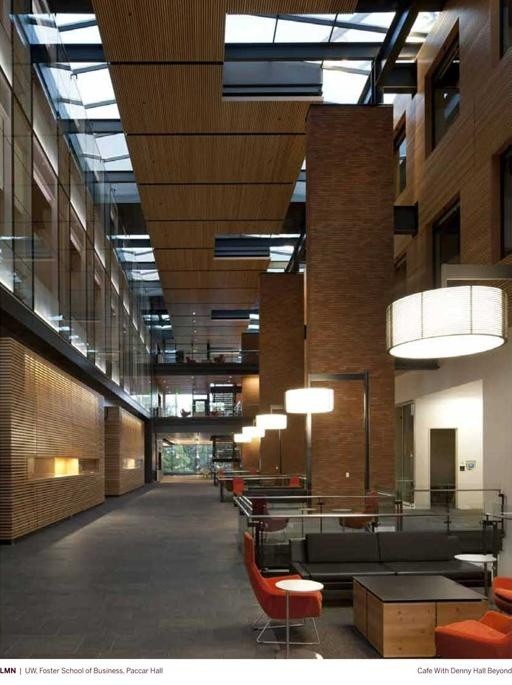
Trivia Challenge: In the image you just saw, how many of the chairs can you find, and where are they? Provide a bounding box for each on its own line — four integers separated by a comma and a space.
433, 572, 512, 659
243, 530, 324, 646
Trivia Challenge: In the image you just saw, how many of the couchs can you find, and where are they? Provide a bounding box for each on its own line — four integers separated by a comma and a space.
286, 524, 498, 599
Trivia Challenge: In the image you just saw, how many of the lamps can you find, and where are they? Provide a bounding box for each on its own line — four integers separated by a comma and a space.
382, 258, 512, 361
